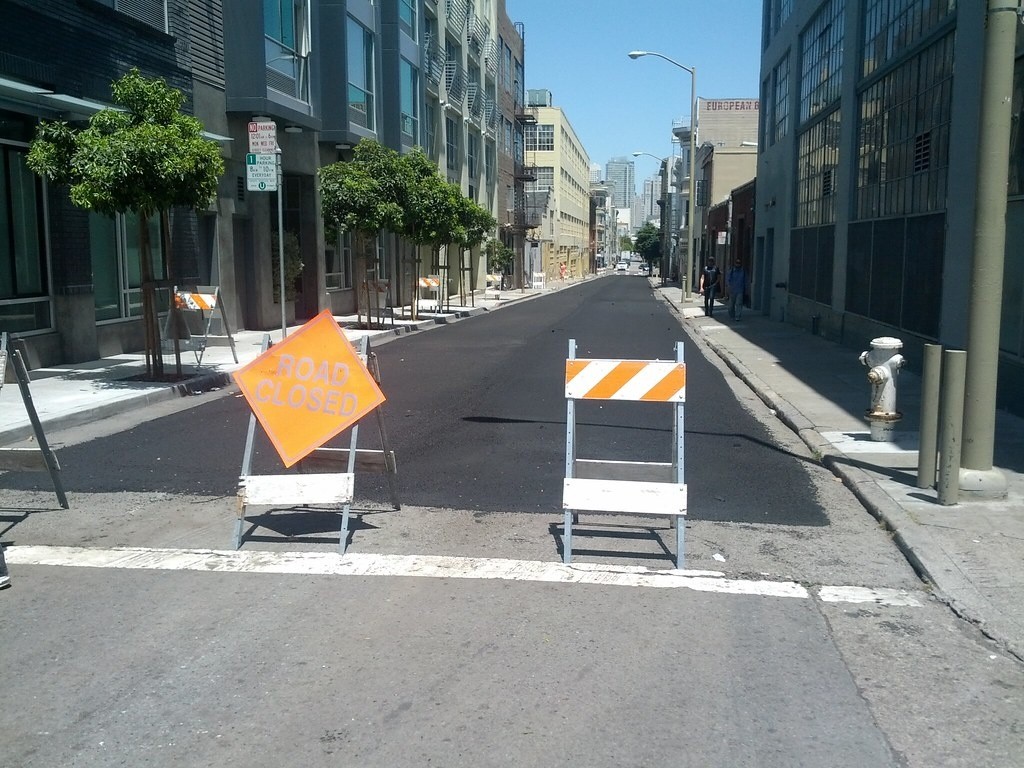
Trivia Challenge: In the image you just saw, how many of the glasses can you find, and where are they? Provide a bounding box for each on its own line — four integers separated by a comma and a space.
709, 259, 714, 262
735, 262, 741, 264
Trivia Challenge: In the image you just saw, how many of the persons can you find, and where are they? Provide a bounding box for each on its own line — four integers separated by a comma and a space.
725, 260, 751, 322
699, 256, 721, 318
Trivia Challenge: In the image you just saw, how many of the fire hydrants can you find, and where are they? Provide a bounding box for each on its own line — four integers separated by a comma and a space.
858, 336, 906, 443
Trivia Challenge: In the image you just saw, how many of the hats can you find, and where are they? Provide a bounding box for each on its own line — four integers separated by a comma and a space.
709, 256, 714, 259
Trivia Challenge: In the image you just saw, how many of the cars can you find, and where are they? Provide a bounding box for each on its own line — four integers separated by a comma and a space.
616, 262, 630, 271
638, 263, 649, 271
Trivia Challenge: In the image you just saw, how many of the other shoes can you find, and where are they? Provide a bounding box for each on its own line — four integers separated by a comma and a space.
735, 318, 739, 321
705, 311, 712, 316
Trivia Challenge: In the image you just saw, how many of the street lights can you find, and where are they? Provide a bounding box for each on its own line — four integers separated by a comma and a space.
632, 151, 667, 285
604, 205, 616, 268
627, 50, 695, 299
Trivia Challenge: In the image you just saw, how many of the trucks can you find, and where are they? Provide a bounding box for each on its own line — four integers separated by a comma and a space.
620, 251, 631, 266
